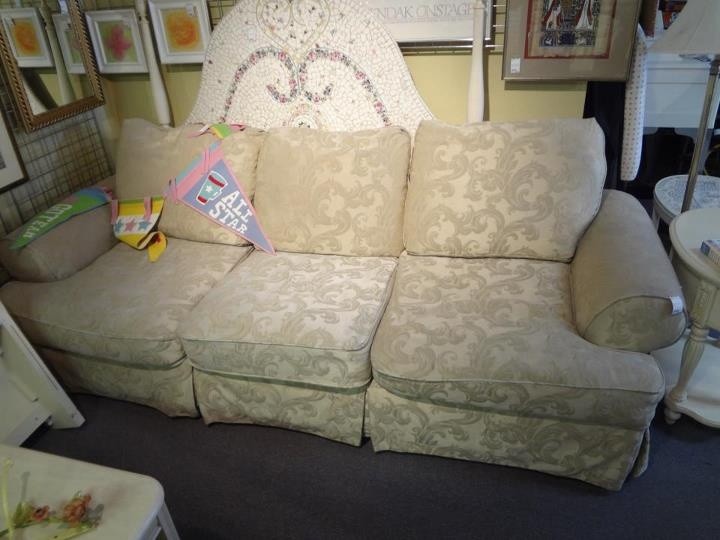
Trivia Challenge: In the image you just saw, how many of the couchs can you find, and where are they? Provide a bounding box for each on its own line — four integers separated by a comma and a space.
0, 117, 690, 494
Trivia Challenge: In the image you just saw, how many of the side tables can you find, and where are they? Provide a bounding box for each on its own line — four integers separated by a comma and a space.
650, 202, 720, 430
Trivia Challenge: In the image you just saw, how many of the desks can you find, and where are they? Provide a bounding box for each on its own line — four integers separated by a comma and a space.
643, 50, 720, 175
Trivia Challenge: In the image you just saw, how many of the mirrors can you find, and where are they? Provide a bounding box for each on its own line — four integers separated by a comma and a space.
0, 0, 107, 134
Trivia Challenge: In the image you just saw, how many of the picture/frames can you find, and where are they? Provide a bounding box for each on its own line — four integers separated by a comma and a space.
501, 0, 642, 81
0, 6, 57, 68
147, 0, 212, 65
83, 7, 149, 74
0, 96, 32, 196
51, 12, 87, 74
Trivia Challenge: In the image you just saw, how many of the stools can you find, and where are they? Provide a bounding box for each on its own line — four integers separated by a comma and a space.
0, 301, 86, 447
651, 175, 720, 263
0, 443, 181, 540
